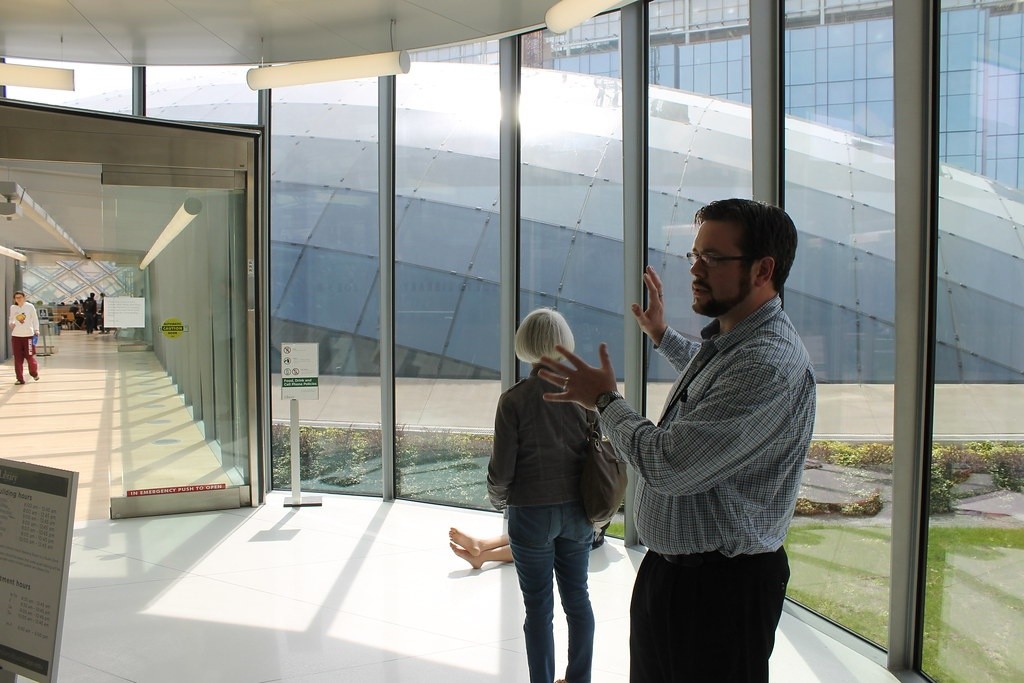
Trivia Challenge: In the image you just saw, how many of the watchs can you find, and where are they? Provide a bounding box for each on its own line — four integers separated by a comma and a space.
591, 391, 623, 410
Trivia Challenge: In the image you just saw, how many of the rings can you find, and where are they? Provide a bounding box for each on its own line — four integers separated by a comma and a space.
563, 376, 569, 389
660, 294, 665, 297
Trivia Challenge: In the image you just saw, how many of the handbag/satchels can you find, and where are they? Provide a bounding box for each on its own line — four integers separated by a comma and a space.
579, 408, 628, 524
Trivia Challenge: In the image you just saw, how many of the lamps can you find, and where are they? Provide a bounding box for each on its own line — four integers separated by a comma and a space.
139, 198, 202, 271
246, 18, 411, 90
0, 245, 27, 261
545, 0, 623, 36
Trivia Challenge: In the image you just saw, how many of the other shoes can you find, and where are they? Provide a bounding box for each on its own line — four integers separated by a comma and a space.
14, 380, 25, 384
34, 376, 40, 381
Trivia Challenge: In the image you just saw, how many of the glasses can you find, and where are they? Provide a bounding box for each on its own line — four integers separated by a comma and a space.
685, 252, 744, 269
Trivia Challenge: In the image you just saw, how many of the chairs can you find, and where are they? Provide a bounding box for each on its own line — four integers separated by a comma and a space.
60, 312, 86, 330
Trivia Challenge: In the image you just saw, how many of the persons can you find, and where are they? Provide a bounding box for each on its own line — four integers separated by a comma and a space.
449, 307, 610, 683
10, 291, 40, 385
534, 198, 816, 683
58, 293, 110, 334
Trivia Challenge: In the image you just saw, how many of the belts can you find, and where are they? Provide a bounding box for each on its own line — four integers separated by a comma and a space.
650, 548, 783, 564
